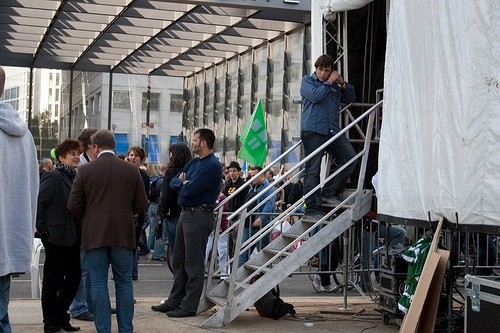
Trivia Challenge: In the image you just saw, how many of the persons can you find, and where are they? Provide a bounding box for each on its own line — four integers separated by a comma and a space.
0, 68, 39, 333
37, 54, 406, 321
35, 138, 82, 333
68, 130, 149, 333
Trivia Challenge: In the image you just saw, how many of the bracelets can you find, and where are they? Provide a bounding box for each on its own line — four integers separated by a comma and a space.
340, 83, 344, 85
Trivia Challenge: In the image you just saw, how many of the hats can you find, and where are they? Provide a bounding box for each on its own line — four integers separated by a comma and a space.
227, 162, 241, 171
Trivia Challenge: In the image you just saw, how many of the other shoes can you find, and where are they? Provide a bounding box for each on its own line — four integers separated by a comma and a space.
138, 250, 150, 255
73, 312, 93, 321
54, 329, 68, 333
321, 197, 346, 205
363, 290, 379, 293
89, 307, 116, 314
152, 257, 167, 261
152, 303, 177, 312
66, 325, 80, 331
167, 307, 196, 317
303, 214, 327, 220
320, 285, 336, 292
312, 280, 321, 293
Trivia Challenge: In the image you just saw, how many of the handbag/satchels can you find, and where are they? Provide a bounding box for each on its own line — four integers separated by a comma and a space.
271, 221, 298, 252
167, 205, 178, 218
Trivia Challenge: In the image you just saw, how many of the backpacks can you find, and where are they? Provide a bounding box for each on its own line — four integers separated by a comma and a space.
147, 176, 164, 200
250, 273, 295, 320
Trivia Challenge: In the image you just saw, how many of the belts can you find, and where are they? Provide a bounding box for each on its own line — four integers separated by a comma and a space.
182, 206, 211, 211
